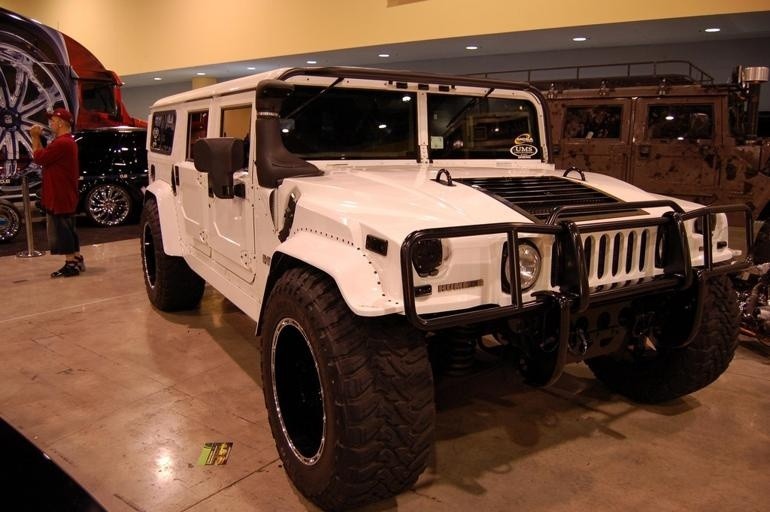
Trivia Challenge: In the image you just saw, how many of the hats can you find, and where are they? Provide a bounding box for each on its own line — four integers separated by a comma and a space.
46, 108, 73, 123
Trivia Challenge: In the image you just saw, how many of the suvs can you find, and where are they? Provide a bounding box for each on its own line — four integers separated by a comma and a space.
537, 79, 768, 228
142, 66, 756, 510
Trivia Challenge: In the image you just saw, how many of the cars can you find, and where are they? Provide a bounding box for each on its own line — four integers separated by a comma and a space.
0, 125, 147, 244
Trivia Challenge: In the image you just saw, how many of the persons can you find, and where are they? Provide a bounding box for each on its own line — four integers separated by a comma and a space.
560, 103, 716, 143
29, 108, 87, 278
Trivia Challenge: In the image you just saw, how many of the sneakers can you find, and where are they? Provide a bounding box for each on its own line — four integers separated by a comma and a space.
51, 255, 85, 278
